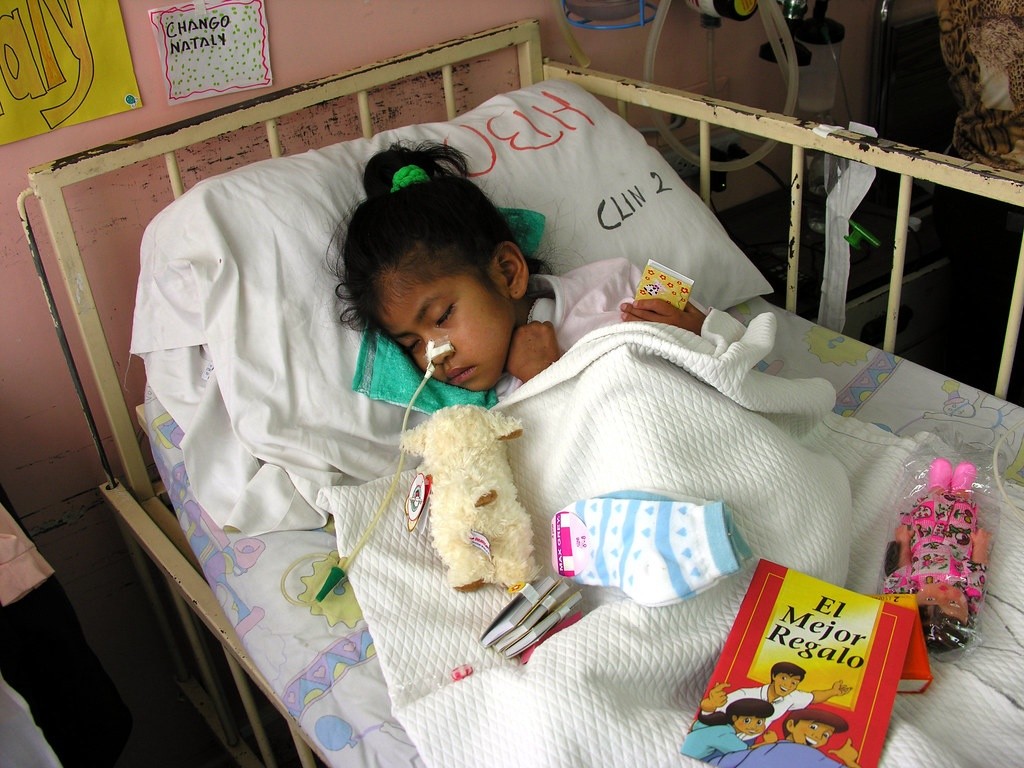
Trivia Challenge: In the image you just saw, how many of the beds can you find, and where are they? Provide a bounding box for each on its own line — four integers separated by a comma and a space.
872, 0, 1024, 295
16, 21, 1024, 768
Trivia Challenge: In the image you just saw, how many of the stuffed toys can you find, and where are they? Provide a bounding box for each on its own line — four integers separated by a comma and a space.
403, 406, 542, 593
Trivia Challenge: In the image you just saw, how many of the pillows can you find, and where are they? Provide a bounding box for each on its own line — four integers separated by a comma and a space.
936, 0, 1024, 174
127, 78, 775, 535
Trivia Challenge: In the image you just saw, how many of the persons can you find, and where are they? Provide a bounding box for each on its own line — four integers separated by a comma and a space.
325, 137, 708, 403
884, 456, 991, 656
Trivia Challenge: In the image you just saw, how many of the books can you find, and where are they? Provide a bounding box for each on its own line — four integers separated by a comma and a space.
683, 558, 935, 768
479, 564, 584, 660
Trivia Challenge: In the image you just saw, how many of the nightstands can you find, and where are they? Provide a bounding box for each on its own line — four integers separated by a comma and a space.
714, 177, 953, 376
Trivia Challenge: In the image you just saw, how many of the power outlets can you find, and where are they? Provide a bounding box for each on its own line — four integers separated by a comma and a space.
667, 133, 740, 184
659, 77, 732, 149
